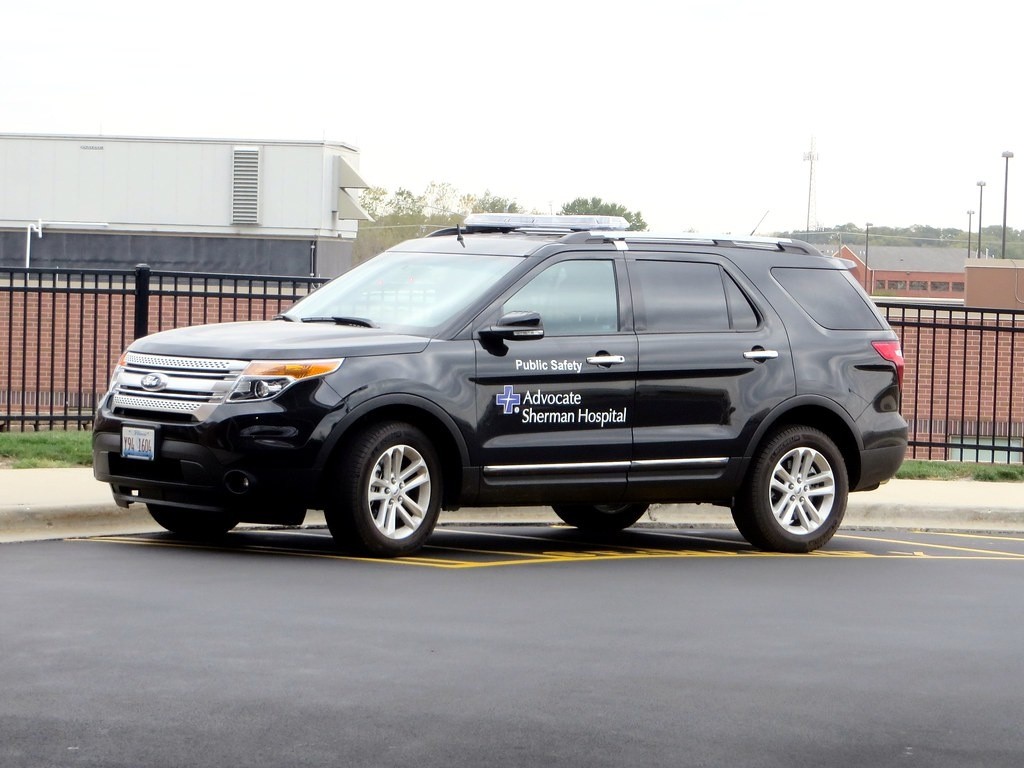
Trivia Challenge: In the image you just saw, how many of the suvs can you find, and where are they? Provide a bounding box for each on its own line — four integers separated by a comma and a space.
91, 212, 910, 557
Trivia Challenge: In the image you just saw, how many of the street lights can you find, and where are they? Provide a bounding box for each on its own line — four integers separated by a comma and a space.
976, 181, 986, 258
863, 222, 873, 291
1001, 151, 1014, 260
967, 210, 975, 258
